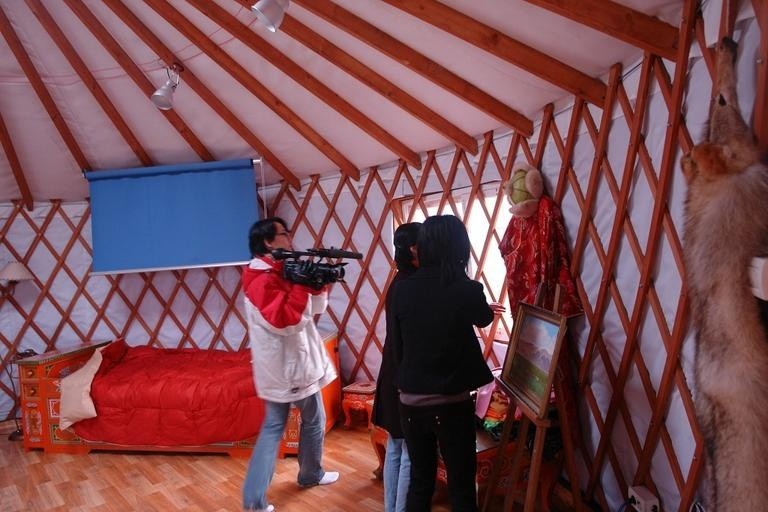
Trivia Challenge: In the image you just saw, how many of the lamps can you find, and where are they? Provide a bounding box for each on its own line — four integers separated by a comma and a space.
150, 63, 185, 111
0, 261, 34, 298
250, 0, 289, 34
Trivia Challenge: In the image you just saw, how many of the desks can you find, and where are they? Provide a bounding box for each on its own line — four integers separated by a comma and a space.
370, 425, 564, 512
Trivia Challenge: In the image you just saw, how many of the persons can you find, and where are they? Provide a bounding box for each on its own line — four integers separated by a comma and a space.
389, 216, 505, 512
370, 222, 424, 512
240, 216, 340, 512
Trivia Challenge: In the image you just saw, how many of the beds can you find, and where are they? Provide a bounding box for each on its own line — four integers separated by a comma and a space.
15, 329, 340, 458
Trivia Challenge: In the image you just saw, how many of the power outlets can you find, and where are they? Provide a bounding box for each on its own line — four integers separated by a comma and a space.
628, 485, 659, 511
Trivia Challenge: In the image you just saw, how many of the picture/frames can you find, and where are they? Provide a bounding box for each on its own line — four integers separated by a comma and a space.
499, 302, 567, 420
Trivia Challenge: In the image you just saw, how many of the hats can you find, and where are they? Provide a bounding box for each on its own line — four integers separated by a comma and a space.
502, 163, 542, 218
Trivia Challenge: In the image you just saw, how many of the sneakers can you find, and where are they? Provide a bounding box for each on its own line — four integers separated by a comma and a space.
255, 505, 275, 512
297, 472, 339, 488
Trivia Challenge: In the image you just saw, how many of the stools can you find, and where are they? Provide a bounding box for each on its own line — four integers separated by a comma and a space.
341, 382, 377, 431
